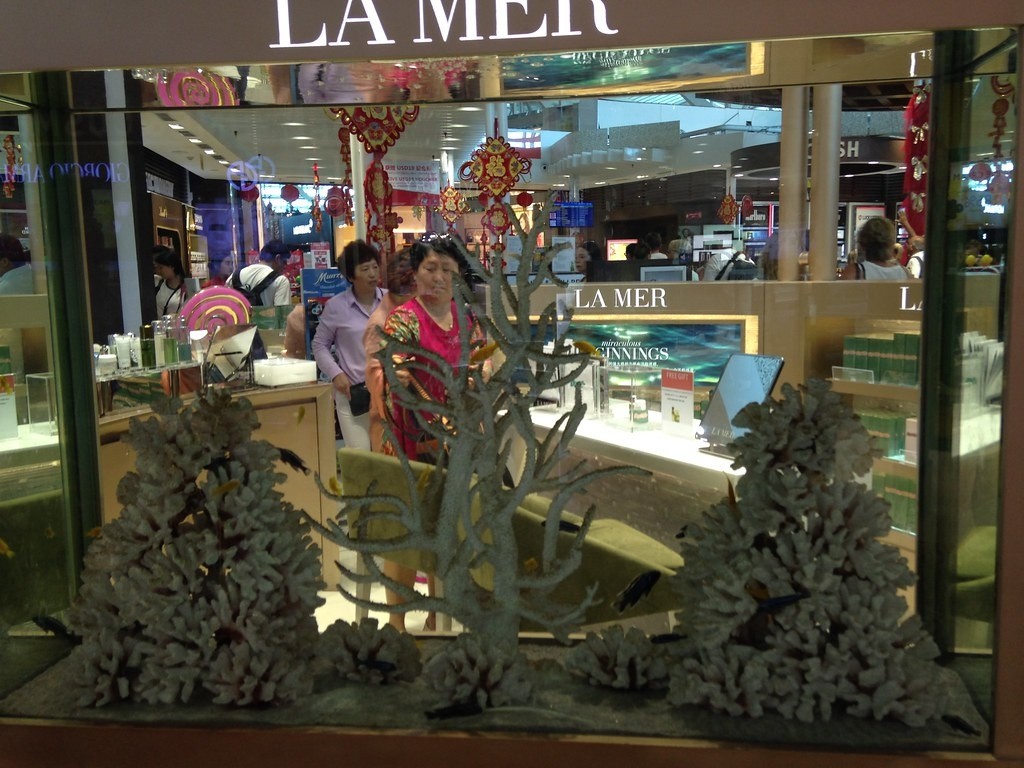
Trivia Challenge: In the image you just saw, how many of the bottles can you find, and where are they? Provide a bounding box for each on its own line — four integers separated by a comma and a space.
592, 348, 609, 414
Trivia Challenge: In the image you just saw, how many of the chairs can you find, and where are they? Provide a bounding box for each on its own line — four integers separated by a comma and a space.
458, 475, 686, 638
335, 446, 454, 634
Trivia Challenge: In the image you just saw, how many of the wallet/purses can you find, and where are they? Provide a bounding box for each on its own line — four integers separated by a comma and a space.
348, 387, 371, 416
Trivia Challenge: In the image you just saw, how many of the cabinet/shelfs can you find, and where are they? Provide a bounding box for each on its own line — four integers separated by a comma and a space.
824, 379, 1001, 552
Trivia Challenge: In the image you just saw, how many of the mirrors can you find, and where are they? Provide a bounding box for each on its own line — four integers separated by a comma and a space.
635, 258, 692, 282
200, 323, 260, 393
693, 353, 786, 462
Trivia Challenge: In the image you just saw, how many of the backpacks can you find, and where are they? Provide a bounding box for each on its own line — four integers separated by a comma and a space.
232, 265, 283, 306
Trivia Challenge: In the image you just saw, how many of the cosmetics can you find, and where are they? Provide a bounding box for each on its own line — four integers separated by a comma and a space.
845, 406, 918, 466
837, 330, 920, 386
871, 474, 918, 536
91, 314, 193, 376
629, 395, 648, 424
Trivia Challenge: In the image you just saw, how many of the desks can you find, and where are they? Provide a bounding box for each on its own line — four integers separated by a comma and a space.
94, 383, 338, 589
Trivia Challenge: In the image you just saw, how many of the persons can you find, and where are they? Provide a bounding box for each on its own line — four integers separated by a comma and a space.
282, 303, 307, 359
311, 238, 391, 453
840, 218, 912, 280
759, 231, 806, 280
894, 209, 925, 280
1, 232, 48, 373
682, 228, 689, 238
151, 245, 188, 323
200, 252, 234, 288
362, 249, 418, 455
624, 226, 757, 281
966, 239, 1002, 272
224, 239, 292, 306
381, 237, 494, 636
575, 242, 603, 282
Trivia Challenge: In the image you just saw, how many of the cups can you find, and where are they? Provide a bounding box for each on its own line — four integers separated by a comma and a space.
633, 410, 648, 423
